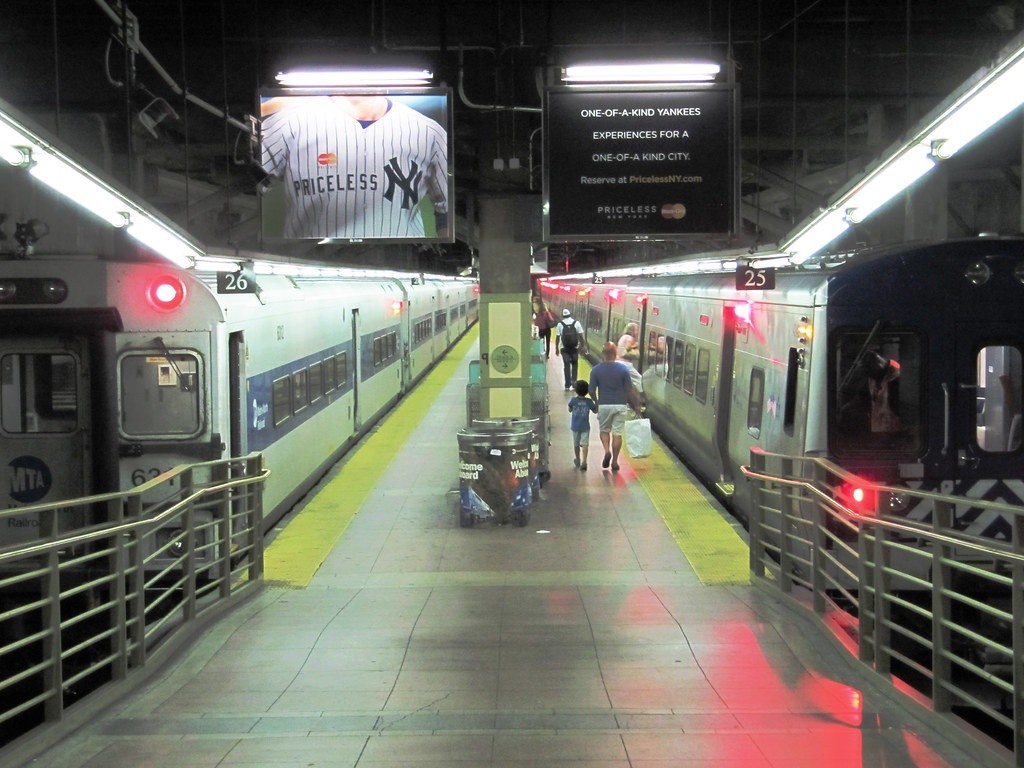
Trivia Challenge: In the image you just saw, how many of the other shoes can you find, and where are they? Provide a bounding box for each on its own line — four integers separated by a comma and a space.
602, 453, 612, 468
574, 458, 581, 467
580, 464, 588, 471
611, 462, 620, 470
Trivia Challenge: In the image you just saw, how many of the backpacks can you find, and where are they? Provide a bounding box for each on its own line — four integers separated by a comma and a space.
560, 320, 579, 350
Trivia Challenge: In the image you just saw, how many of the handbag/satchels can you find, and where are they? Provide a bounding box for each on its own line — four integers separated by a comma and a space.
546, 318, 557, 328
625, 413, 652, 459
621, 347, 641, 360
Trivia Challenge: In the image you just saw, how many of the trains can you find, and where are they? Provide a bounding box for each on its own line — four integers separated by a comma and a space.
534, 229, 1024, 592
0, 216, 481, 581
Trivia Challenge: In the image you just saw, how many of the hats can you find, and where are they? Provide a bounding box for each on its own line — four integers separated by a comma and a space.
562, 309, 571, 316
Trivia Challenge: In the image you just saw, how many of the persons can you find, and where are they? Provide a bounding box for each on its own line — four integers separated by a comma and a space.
260, 94, 449, 239
616, 323, 646, 412
534, 302, 554, 361
555, 309, 584, 392
876, 348, 901, 430
589, 342, 641, 470
569, 380, 598, 469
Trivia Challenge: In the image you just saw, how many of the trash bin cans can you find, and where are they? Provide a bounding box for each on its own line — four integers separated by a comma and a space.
456, 416, 547, 527
